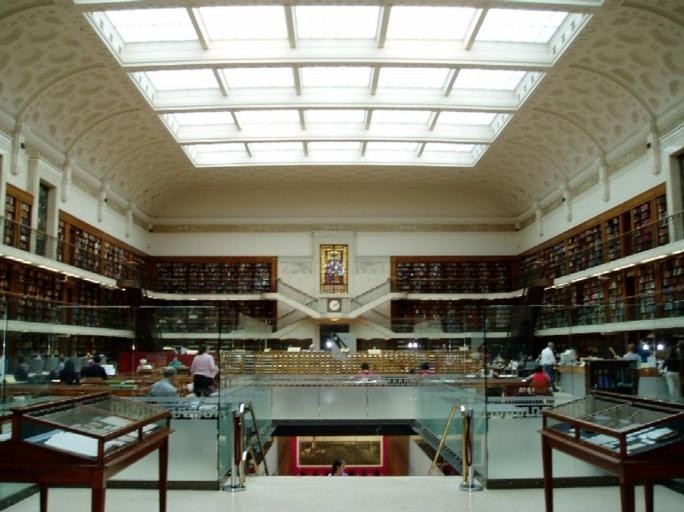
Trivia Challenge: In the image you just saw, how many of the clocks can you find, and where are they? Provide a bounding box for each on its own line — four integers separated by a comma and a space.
327, 299, 342, 312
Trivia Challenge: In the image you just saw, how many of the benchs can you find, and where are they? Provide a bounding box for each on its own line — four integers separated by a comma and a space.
443, 368, 553, 419
1, 369, 190, 399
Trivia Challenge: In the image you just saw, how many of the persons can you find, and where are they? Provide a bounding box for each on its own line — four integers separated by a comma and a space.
328, 458, 348, 477
13, 349, 108, 386
416, 361, 435, 375
621, 337, 684, 379
149, 366, 178, 397
207, 372, 222, 396
542, 339, 559, 395
166, 355, 185, 369
356, 362, 374, 375
189, 344, 216, 397
531, 363, 553, 394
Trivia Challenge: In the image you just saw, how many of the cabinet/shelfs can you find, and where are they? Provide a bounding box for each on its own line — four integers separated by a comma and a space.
391, 299, 515, 333
537, 391, 684, 512
5, 183, 148, 292
515, 252, 684, 331
2, 330, 130, 372
515, 327, 684, 358
391, 255, 513, 292
148, 255, 278, 295
139, 300, 278, 333
515, 183, 667, 294
0, 392, 176, 512
391, 338, 514, 357
1, 257, 148, 331
145, 340, 277, 374
